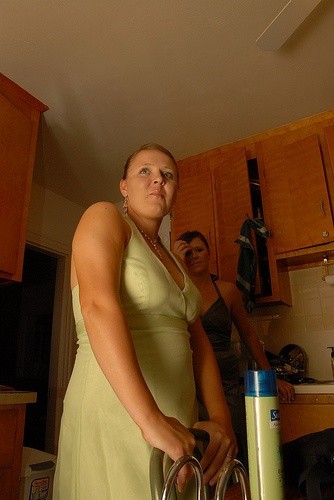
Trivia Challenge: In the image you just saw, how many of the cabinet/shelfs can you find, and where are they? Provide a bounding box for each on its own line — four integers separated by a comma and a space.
166, 111, 334, 309
0, 403, 26, 500
277, 394, 334, 443
0, 68, 50, 285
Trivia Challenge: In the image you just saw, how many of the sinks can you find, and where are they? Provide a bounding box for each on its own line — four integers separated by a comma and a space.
294, 380, 334, 394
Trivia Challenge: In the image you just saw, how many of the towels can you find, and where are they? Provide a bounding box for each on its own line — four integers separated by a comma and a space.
234, 218, 269, 313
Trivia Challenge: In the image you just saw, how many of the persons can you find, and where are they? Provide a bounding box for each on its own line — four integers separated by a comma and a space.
172, 231, 295, 457
52, 143, 239, 500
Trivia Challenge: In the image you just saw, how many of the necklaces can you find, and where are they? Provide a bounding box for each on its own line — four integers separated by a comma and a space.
137, 225, 161, 257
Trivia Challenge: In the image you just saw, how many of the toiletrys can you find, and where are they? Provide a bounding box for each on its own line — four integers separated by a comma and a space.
327, 347, 334, 379
